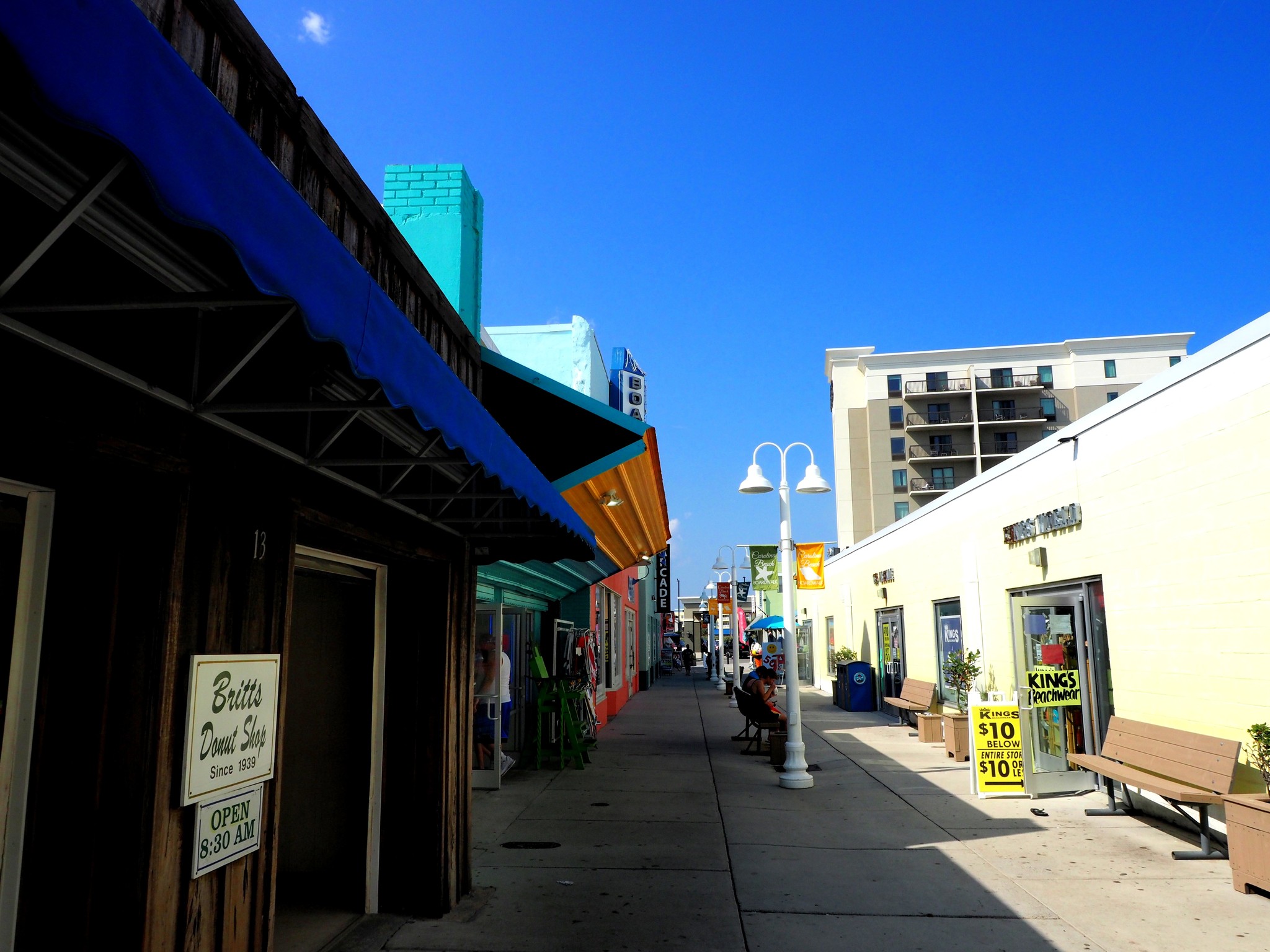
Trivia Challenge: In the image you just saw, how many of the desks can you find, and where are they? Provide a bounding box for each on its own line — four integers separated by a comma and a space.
941, 453, 947, 456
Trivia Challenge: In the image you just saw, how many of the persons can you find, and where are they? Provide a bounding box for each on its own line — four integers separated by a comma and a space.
742, 665, 787, 743
681, 644, 694, 676
473, 634, 516, 778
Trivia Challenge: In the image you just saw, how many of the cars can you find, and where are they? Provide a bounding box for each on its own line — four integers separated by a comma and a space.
674, 640, 684, 651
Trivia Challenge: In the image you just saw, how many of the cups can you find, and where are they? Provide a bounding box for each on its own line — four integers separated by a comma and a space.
773, 687, 778, 695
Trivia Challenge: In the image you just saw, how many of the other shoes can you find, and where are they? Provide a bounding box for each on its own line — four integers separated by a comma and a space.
686, 673, 691, 676
500, 755, 516, 776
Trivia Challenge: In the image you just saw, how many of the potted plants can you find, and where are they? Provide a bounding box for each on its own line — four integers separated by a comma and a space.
941, 648, 989, 762
1219, 722, 1270, 894
828, 646, 858, 705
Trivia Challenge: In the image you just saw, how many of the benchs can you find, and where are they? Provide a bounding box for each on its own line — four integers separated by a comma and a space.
882, 677, 937, 737
1067, 716, 1242, 863
722, 666, 779, 755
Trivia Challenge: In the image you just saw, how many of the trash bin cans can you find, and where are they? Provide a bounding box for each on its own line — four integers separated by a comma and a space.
835, 659, 874, 712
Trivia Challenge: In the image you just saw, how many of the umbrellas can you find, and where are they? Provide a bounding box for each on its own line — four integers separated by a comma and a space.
750, 615, 801, 668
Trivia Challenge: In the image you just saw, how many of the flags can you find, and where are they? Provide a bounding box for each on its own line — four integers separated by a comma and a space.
795, 542, 826, 590
708, 599, 718, 615
737, 582, 751, 602
723, 599, 733, 614
665, 612, 675, 633
750, 544, 780, 590
717, 582, 731, 603
737, 607, 749, 646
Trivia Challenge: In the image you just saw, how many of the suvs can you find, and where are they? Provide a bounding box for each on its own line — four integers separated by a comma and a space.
716, 636, 757, 658
700, 637, 708, 653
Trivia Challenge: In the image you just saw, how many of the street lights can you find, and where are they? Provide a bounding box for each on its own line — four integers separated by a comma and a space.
739, 442, 831, 789
712, 545, 751, 708
704, 571, 732, 691
698, 584, 719, 681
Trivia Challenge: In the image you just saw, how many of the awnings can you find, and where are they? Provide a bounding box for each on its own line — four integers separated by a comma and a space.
744, 614, 762, 631
664, 633, 679, 636
0, 0, 598, 566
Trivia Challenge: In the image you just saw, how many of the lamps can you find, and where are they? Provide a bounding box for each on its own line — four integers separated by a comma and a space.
1029, 547, 1048, 566
599, 489, 624, 506
801, 608, 807, 614
637, 551, 650, 560
877, 587, 887, 598
652, 595, 656, 601
1057, 436, 1078, 443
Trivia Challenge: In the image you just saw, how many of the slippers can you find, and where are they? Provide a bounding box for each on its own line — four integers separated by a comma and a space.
764, 740, 770, 743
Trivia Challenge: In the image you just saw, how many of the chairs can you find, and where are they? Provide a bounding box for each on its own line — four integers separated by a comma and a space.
950, 449, 958, 455
940, 414, 969, 423
930, 449, 938, 456
1015, 380, 1037, 387
913, 482, 934, 491
942, 384, 966, 390
996, 413, 1028, 420
